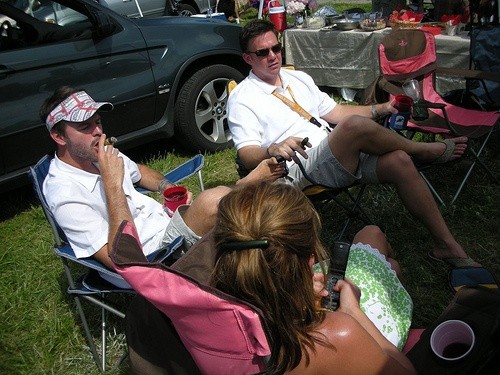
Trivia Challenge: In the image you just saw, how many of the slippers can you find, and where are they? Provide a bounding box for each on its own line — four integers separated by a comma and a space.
428, 251, 482, 275
413, 136, 469, 172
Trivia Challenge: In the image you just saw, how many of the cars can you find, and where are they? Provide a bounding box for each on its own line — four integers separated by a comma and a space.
0, 0, 218, 38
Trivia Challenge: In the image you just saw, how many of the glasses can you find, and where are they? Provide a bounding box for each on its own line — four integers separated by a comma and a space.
243, 42, 282, 57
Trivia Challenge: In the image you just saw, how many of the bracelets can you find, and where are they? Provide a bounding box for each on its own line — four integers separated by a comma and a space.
371, 103, 383, 121
155, 175, 172, 195
265, 142, 276, 158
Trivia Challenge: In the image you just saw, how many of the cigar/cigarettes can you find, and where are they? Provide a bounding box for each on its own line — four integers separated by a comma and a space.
299, 137, 309, 147
103, 137, 118, 145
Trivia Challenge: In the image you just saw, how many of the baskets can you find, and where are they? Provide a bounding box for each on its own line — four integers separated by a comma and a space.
390, 11, 422, 30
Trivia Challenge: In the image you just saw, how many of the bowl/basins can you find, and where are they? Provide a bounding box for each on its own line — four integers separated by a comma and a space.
390, 19, 420, 29
306, 12, 387, 30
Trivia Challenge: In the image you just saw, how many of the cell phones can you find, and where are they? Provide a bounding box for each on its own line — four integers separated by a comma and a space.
320, 241, 351, 310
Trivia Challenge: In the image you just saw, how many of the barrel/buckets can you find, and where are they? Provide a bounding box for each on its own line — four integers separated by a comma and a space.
267, 0, 286, 31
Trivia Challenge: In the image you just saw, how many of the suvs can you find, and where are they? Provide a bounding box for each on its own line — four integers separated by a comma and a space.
0, 0, 255, 186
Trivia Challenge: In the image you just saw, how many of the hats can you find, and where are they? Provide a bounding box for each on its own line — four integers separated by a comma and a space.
45, 90, 114, 132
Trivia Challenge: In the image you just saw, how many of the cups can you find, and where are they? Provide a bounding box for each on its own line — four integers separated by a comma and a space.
430, 320, 475, 361
395, 94, 414, 113
446, 23, 458, 35
402, 80, 422, 102
162, 186, 187, 217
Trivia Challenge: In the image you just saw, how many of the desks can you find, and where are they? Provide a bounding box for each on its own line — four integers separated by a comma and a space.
285, 26, 469, 95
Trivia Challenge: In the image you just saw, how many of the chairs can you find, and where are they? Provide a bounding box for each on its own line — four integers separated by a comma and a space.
30, 27, 500, 375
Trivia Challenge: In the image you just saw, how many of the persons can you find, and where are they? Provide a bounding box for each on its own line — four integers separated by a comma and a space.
226, 19, 483, 267
211, 181, 419, 375
40, 83, 289, 289
